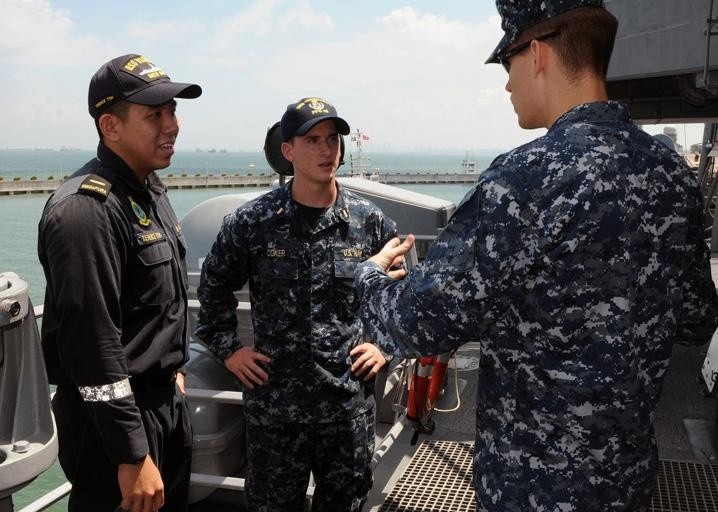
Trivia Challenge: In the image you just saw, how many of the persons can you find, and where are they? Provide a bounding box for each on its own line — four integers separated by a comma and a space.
354, 1, 718, 511
194, 99, 407, 511
37, 54, 202, 512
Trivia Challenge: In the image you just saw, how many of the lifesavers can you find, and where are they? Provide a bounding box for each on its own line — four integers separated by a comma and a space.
407, 354, 451, 421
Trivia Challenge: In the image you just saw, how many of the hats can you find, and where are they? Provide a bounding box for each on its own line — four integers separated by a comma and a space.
281, 98, 350, 142
88, 54, 202, 118
485, 0, 605, 64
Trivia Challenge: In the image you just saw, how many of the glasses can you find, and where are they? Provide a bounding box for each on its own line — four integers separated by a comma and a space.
499, 31, 557, 73
